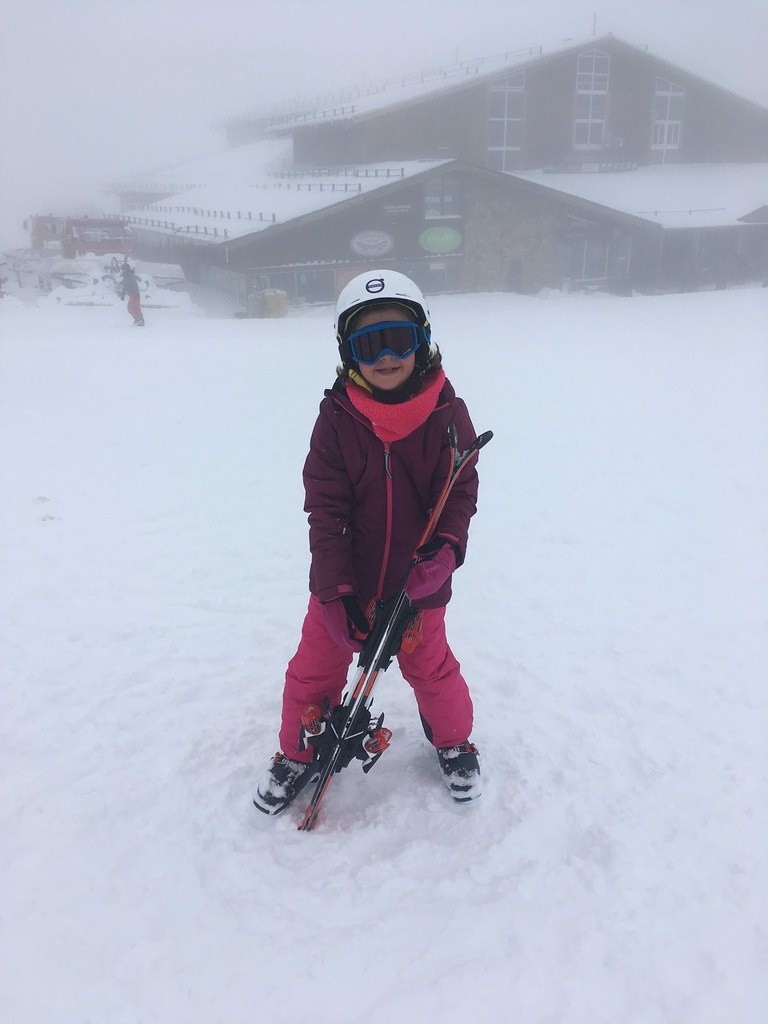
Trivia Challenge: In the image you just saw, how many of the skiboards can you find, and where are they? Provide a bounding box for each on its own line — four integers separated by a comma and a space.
296, 422, 496, 837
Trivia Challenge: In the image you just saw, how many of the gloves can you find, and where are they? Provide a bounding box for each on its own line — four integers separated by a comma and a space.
318, 585, 370, 654
405, 539, 456, 599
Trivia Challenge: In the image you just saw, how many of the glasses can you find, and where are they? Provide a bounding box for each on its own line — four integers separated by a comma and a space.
348, 321, 423, 367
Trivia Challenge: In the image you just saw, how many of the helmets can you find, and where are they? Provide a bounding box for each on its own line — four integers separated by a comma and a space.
333, 270, 432, 342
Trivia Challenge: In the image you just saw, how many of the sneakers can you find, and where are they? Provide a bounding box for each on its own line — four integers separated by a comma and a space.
133, 320, 144, 326
251, 754, 322, 818
438, 739, 483, 803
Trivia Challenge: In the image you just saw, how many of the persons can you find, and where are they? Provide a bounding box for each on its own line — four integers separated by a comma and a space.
252, 267, 494, 817
114, 263, 146, 326
